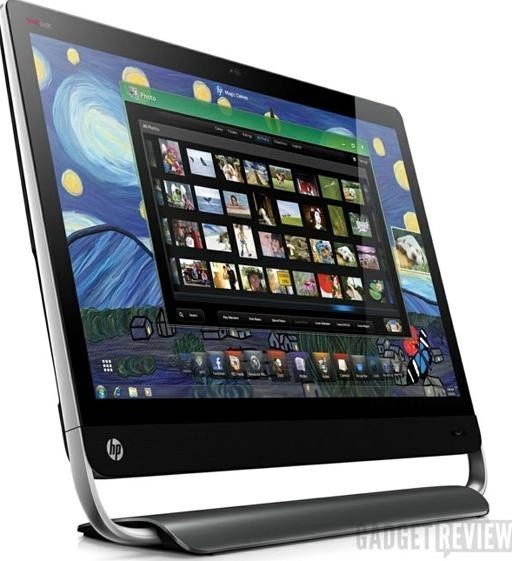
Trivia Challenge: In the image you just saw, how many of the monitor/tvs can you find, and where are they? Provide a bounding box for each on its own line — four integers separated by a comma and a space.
0, 0, 490, 555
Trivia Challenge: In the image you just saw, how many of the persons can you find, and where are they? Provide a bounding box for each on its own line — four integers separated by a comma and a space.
158, 137, 374, 303
